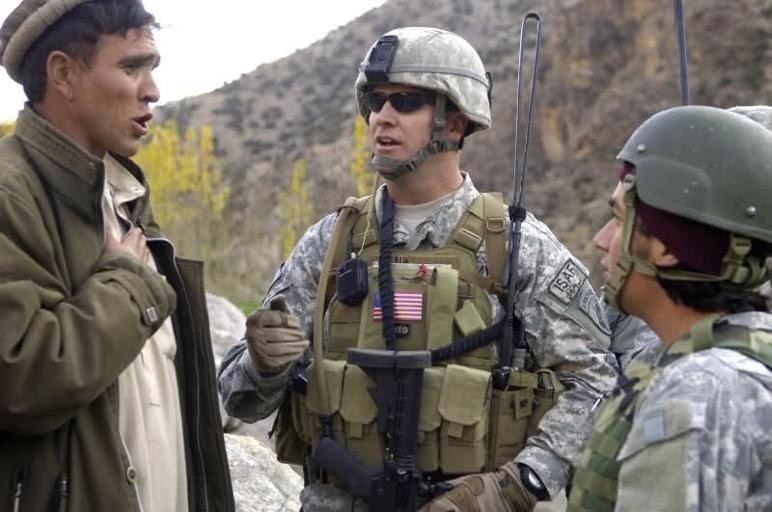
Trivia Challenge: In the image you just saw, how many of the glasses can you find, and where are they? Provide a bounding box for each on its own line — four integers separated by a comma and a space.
363, 91, 438, 114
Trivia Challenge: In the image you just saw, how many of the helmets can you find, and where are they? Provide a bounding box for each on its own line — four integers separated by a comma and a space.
616, 106, 771, 243
355, 27, 492, 133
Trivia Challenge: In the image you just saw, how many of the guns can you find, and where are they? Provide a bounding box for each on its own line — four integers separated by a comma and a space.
313, 346, 456, 512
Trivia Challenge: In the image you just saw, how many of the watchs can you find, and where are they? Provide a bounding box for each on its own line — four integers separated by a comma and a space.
518, 463, 549, 501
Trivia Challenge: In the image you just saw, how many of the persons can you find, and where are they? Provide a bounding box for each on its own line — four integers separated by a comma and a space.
0, 0, 235, 512
564, 105, 772, 512
221, 28, 618, 512
611, 105, 772, 369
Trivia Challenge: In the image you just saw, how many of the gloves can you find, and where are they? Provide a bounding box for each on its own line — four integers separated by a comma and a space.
245, 295, 310, 373
419, 461, 538, 512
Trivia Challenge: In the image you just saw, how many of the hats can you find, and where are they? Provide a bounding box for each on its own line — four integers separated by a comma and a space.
0, 0, 83, 84
